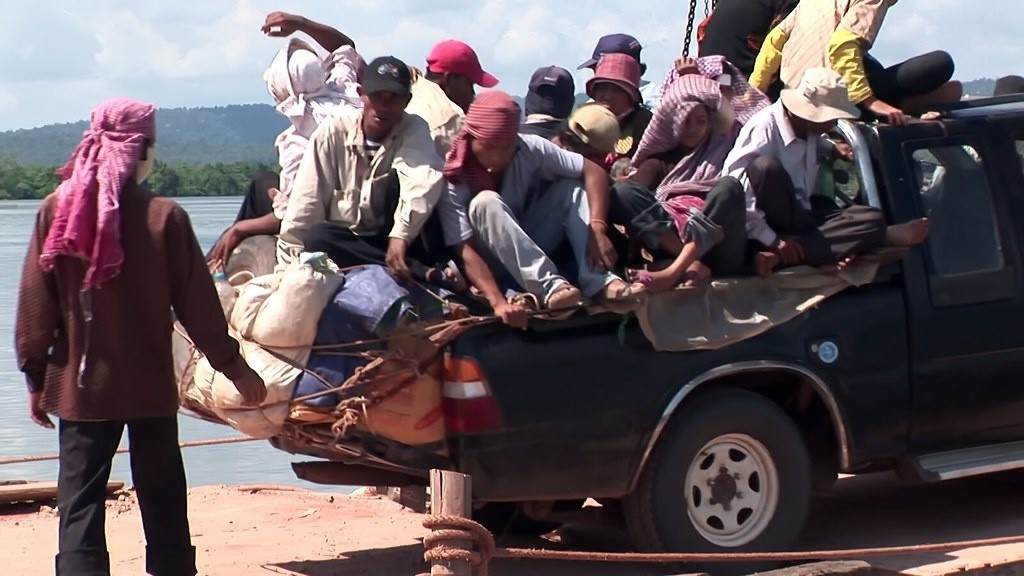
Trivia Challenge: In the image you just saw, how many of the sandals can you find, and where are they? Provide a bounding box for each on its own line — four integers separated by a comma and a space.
625, 268, 657, 294
674, 272, 708, 289
432, 259, 465, 290
548, 283, 582, 308
599, 279, 647, 301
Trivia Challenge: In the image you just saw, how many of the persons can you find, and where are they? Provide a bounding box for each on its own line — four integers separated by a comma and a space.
438, 90, 650, 329
204, 0, 964, 297
14, 95, 268, 576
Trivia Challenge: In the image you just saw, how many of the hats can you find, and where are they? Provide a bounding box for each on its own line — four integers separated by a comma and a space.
428, 38, 499, 88
586, 54, 644, 107
362, 56, 411, 95
577, 31, 647, 73
779, 65, 861, 122
529, 64, 575, 98
568, 105, 620, 154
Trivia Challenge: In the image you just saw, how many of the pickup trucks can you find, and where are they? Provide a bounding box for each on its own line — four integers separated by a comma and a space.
266, 93, 1024, 576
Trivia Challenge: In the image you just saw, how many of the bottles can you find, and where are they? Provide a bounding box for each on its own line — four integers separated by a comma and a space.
213, 273, 236, 312
